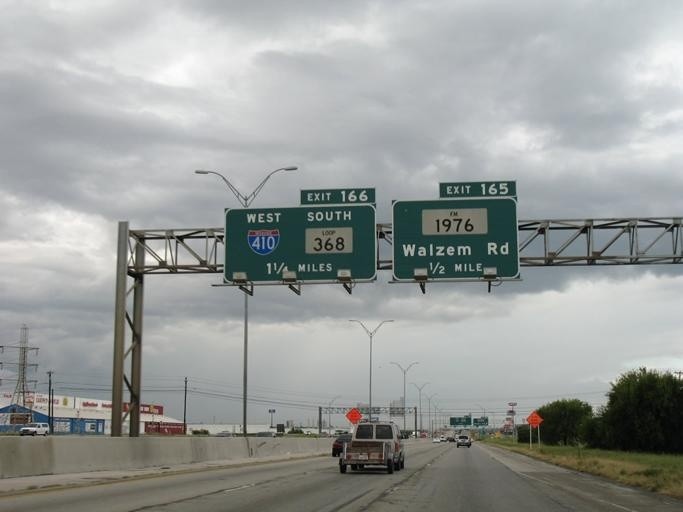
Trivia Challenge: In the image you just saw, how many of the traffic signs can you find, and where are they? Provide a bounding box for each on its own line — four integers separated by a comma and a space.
224, 206, 377, 285
302, 189, 378, 205
391, 198, 521, 282
438, 180, 518, 199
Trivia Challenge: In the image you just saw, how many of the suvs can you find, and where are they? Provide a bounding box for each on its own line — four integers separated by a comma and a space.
457, 435, 471, 448
19, 422, 50, 436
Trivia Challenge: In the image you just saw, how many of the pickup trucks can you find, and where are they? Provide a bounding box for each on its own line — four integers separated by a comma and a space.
339, 420, 406, 474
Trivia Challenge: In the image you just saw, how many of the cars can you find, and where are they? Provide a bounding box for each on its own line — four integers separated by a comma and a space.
399, 428, 476, 443
332, 433, 354, 457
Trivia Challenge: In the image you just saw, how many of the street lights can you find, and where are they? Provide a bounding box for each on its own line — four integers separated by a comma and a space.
410, 380, 446, 436
349, 318, 394, 423
508, 402, 517, 444
268, 409, 276, 427
389, 360, 420, 432
46, 370, 55, 424
193, 166, 302, 438
